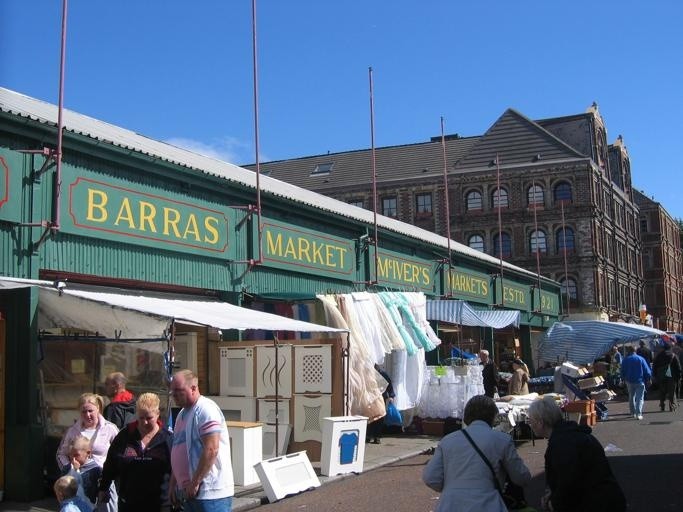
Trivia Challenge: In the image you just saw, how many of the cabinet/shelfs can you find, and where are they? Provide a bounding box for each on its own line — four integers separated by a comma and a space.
320, 415, 369, 477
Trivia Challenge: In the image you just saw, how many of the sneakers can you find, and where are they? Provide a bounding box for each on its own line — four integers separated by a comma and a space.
631, 414, 636, 418
374, 440, 380, 444
366, 439, 370, 443
637, 416, 643, 420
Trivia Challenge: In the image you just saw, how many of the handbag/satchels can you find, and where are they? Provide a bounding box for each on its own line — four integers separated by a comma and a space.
500, 481, 527, 511
665, 364, 672, 378
384, 402, 403, 427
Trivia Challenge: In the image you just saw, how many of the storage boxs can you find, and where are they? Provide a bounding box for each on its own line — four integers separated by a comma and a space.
564, 401, 590, 414
580, 412, 592, 425
576, 399, 595, 412
591, 411, 596, 425
420, 420, 444, 435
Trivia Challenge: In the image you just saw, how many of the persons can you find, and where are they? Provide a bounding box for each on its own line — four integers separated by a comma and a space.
98, 393, 174, 512
526, 397, 627, 512
612, 338, 683, 420
479, 350, 499, 398
56, 392, 120, 512
515, 355, 531, 377
366, 365, 396, 444
63, 435, 111, 503
54, 476, 92, 512
422, 395, 531, 512
168, 370, 234, 512
103, 372, 137, 431
508, 359, 529, 395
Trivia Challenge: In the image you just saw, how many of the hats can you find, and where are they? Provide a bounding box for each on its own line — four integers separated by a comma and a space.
675, 335, 683, 343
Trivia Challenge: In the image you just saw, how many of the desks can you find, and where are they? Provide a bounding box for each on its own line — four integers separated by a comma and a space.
528, 375, 554, 392
492, 409, 541, 446
226, 421, 263, 487
